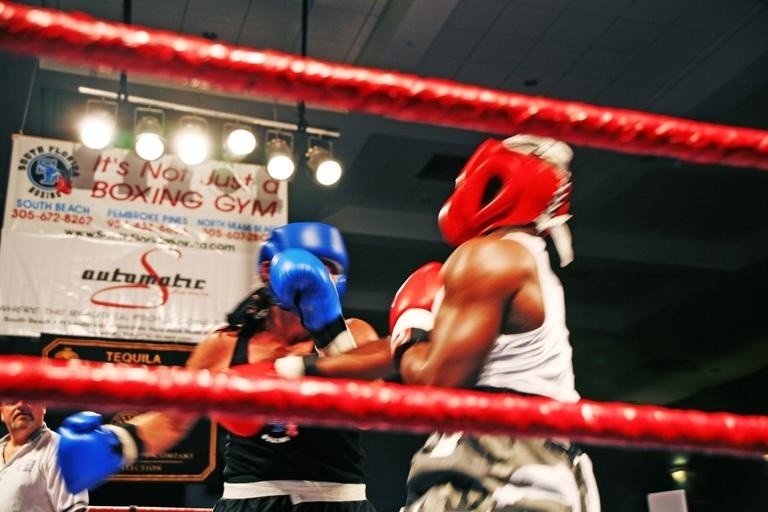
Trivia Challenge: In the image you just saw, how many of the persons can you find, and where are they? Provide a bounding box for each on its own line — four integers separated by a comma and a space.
0, 396, 89, 512
56, 221, 394, 512
209, 134, 602, 511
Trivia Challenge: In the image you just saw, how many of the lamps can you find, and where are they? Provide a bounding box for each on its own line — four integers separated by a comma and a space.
78, 96, 344, 194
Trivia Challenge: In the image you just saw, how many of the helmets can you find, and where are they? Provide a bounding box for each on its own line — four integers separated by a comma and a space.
257, 221, 348, 310
438, 133, 574, 245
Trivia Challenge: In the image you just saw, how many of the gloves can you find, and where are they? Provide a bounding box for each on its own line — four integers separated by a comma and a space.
270, 248, 346, 348
205, 353, 320, 438
56, 411, 144, 496
389, 262, 447, 369
